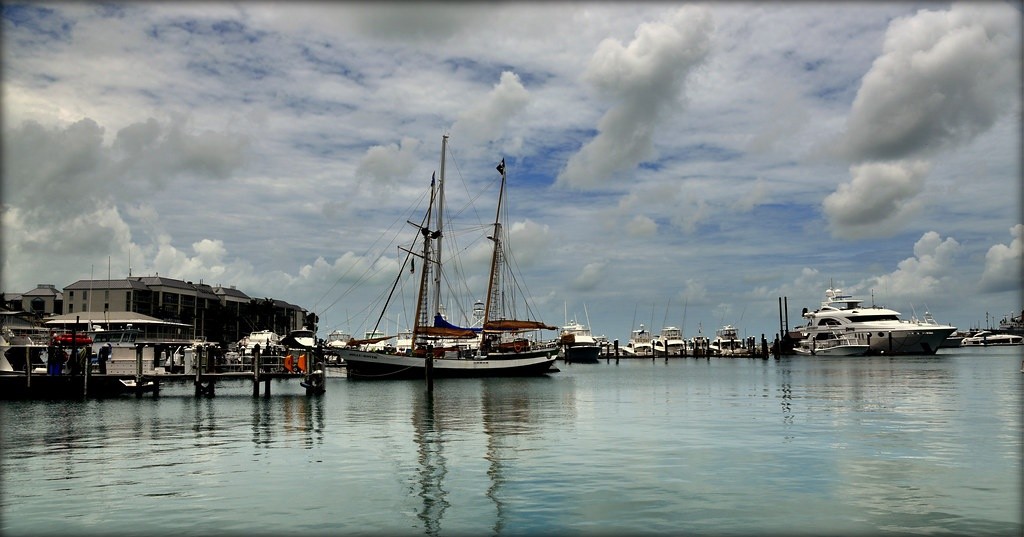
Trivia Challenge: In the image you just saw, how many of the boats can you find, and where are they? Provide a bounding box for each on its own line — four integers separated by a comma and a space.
960, 331, 1023, 346
792, 288, 957, 357
939, 337, 963, 351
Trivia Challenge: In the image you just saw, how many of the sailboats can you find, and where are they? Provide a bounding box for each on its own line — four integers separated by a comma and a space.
330, 131, 563, 376
559, 294, 792, 360
